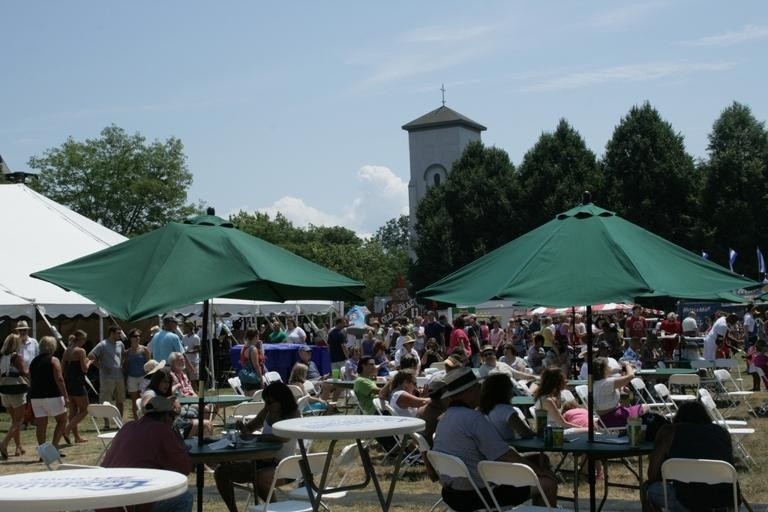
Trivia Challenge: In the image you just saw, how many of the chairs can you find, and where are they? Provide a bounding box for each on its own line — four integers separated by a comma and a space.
558, 382, 590, 415
662, 459, 739, 512
426, 450, 491, 512
477, 460, 551, 512
631, 358, 760, 471
228, 369, 401, 421
87, 397, 143, 464
243, 441, 366, 512
39, 442, 101, 470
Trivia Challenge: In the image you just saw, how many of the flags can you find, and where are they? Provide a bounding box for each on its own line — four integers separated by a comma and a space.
756, 247, 766, 274
728, 247, 737, 269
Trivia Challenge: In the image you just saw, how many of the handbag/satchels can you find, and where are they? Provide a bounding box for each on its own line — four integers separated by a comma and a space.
238, 362, 259, 385
0, 370, 31, 395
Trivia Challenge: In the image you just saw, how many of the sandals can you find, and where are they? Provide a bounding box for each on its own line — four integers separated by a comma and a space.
1, 432, 90, 462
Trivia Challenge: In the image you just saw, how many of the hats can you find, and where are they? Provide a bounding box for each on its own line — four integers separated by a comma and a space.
149, 325, 161, 337
440, 366, 485, 399
479, 343, 498, 356
142, 395, 176, 413
578, 345, 600, 358
443, 354, 465, 368
142, 359, 167, 380
14, 320, 31, 330
402, 335, 416, 346
297, 344, 313, 352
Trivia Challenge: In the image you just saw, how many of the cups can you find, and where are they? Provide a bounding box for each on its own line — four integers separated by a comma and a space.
535, 408, 548, 437
340, 366, 345, 378
627, 418, 640, 450
331, 368, 339, 382
619, 393, 629, 407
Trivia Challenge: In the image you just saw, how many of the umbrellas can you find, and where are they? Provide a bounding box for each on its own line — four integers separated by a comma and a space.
620, 294, 754, 367
30, 205, 369, 511
415, 189, 764, 511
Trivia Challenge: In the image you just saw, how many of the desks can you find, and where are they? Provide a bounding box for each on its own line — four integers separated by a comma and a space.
1, 469, 189, 512
509, 395, 537, 423
272, 415, 428, 512
175, 395, 253, 436
229, 342, 334, 388
508, 434, 654, 511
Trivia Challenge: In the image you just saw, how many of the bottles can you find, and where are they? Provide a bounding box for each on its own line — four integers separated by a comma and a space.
543, 425, 564, 446
